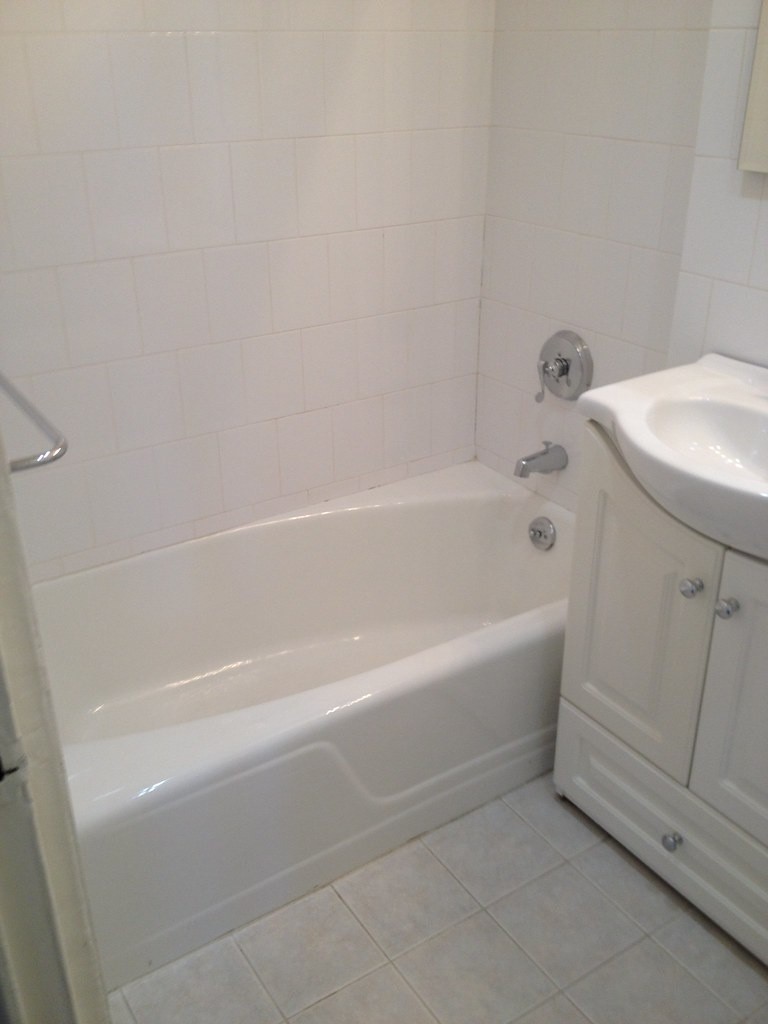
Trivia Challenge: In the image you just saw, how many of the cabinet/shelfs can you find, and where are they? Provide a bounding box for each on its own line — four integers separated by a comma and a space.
552, 421, 767, 968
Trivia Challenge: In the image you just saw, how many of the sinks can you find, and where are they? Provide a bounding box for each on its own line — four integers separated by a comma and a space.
576, 352, 768, 565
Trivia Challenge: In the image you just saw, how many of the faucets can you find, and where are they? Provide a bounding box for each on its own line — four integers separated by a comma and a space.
514, 443, 568, 479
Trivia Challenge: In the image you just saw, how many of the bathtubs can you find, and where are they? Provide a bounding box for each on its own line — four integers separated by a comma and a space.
28, 460, 572, 869
